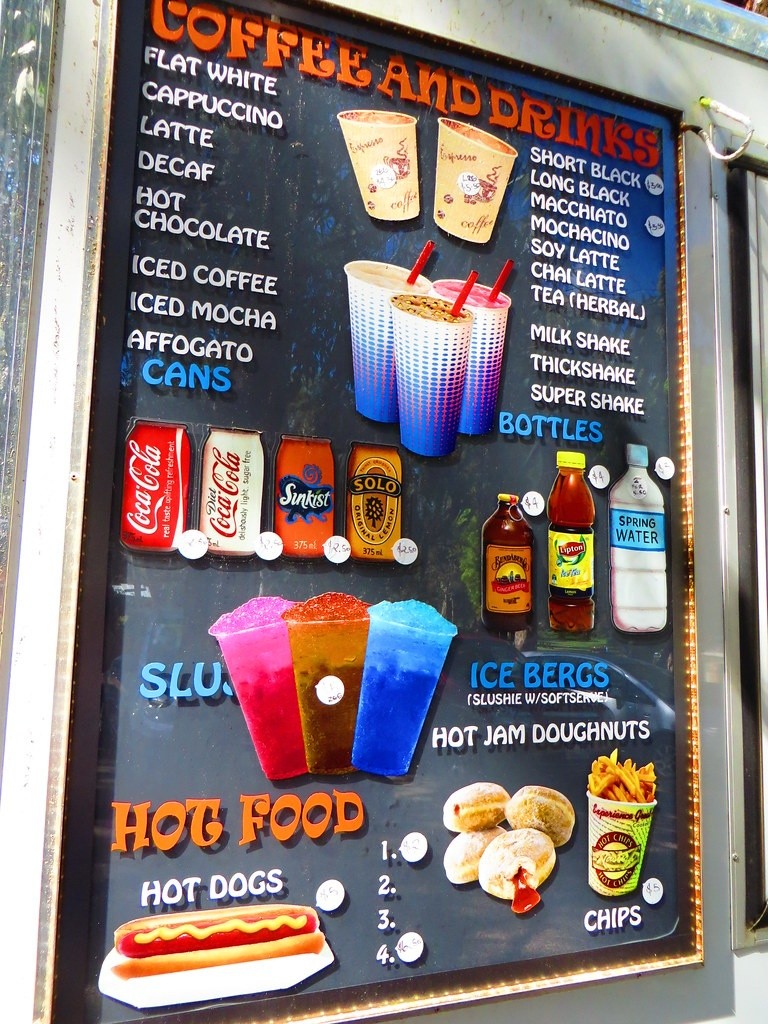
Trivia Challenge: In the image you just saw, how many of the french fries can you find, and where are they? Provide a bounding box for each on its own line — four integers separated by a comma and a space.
587, 748, 657, 803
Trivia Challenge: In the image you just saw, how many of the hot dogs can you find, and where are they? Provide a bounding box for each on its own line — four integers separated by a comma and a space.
109, 904, 325, 981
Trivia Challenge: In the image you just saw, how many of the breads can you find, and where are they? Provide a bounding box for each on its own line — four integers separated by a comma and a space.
443, 781, 576, 914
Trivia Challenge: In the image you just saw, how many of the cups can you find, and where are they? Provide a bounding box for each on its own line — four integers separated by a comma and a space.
435, 117, 518, 243
207, 617, 308, 781
586, 789, 657, 897
285, 615, 371, 775
343, 260, 432, 424
336, 108, 421, 221
389, 293, 475, 457
351, 616, 455, 776
432, 280, 510, 434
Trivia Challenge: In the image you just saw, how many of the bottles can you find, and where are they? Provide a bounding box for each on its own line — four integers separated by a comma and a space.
480, 494, 535, 631
609, 444, 667, 633
548, 452, 595, 631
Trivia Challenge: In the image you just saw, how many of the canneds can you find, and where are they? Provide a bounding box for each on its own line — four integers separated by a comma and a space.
273, 434, 334, 558
344, 440, 402, 563
198, 427, 265, 556
119, 418, 193, 553
